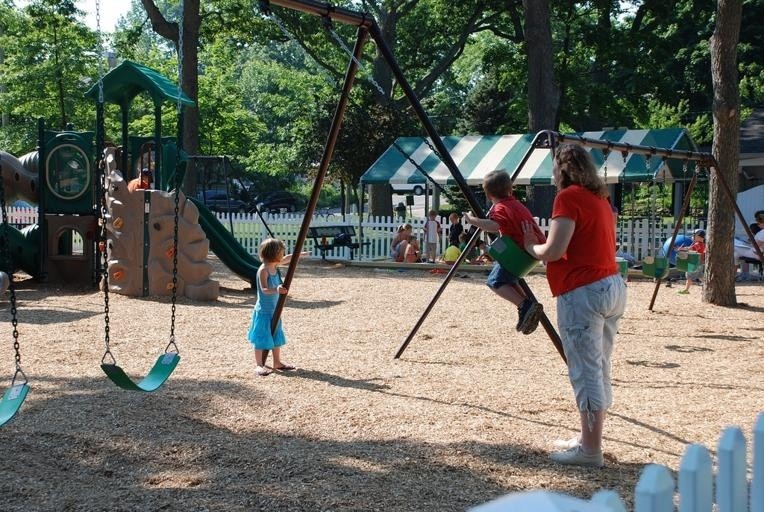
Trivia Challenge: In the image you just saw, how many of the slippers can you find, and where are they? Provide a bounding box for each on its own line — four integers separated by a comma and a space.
272, 364, 295, 369
256, 366, 267, 375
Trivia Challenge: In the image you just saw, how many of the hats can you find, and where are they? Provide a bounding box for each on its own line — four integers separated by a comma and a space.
143, 168, 153, 183
693, 230, 705, 237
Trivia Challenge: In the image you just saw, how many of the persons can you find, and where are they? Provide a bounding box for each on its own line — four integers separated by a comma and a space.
125, 168, 152, 196
249, 238, 313, 373
390, 209, 496, 265
523, 141, 628, 465
460, 169, 544, 337
752, 206, 763, 257
735, 221, 761, 280
676, 227, 706, 294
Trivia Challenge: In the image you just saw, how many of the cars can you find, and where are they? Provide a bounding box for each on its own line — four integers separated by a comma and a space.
188, 175, 306, 218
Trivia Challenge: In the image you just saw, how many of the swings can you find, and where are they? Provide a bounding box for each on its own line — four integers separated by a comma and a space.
96, 0, 186, 392
603, 149, 628, 276
267, 10, 540, 275
0, 182, 29, 428
642, 155, 667, 276
676, 159, 702, 272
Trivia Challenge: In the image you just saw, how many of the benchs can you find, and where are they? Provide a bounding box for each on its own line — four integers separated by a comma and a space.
306, 225, 370, 260
741, 257, 764, 274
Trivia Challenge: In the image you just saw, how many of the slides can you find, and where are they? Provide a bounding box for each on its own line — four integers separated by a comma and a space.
191, 198, 263, 281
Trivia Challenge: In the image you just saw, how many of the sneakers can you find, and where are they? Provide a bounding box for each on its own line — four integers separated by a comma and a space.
736, 271, 749, 281
679, 289, 689, 293
516, 299, 543, 335
550, 446, 604, 466
554, 436, 581, 449
666, 281, 671, 287
